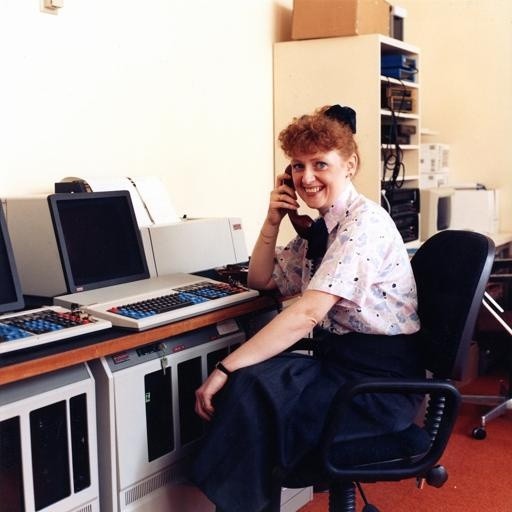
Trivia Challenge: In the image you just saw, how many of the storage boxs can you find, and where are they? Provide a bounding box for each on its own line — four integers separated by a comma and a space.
288, 0, 392, 44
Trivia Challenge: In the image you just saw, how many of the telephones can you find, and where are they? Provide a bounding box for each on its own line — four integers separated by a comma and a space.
283, 164, 316, 241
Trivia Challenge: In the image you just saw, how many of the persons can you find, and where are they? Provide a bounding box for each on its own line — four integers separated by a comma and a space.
189, 115, 428, 512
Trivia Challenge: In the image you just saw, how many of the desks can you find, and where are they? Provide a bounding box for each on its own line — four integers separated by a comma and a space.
1, 290, 302, 390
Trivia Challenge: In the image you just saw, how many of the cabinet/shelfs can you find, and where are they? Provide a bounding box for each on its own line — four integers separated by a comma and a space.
272, 32, 420, 245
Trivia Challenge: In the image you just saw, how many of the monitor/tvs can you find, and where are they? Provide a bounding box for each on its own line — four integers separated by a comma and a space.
47, 190, 151, 293
0, 201, 25, 314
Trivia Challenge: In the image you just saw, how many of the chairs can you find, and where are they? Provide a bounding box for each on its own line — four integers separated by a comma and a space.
447, 237, 512, 441
278, 228, 497, 511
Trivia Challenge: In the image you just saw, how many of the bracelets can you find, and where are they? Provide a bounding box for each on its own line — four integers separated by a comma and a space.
214, 363, 233, 378
260, 230, 277, 244
